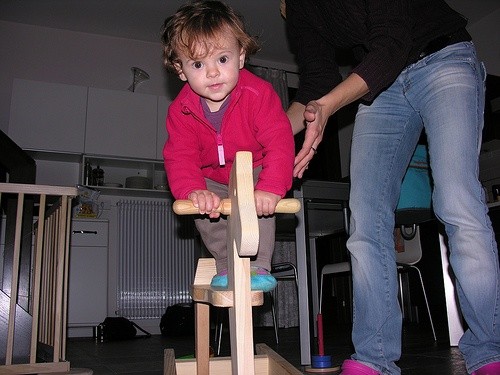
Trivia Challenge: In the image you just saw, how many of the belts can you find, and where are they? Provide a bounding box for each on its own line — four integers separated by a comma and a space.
413, 28, 472, 61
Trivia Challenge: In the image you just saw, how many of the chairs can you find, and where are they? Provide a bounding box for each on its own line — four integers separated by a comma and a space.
316, 226, 439, 349
215, 213, 298, 356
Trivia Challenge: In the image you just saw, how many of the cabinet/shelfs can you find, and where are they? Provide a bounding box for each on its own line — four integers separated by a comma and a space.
71, 219, 108, 342
81, 154, 168, 191
10, 83, 166, 163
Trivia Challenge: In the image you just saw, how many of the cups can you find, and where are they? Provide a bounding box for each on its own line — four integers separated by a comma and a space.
492, 185, 500, 202
155, 173, 169, 190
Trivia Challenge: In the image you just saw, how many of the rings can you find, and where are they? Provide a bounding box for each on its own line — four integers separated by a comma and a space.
311, 146, 317, 152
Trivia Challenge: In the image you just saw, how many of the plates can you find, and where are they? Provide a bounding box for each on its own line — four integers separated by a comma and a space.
103, 183, 123, 187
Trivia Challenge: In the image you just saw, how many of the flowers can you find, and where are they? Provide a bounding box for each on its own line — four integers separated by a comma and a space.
79, 190, 95, 202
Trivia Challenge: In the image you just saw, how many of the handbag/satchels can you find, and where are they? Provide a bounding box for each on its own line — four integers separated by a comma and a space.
160, 304, 218, 343
97, 317, 152, 343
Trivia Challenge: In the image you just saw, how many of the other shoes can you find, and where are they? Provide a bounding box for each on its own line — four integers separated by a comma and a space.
339, 359, 382, 375
210, 270, 228, 289
472, 361, 500, 375
251, 268, 278, 292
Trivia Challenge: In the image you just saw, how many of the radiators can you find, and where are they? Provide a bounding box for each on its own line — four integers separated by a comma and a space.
117, 199, 192, 318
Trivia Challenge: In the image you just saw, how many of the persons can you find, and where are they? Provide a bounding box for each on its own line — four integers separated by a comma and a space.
285, 0, 500, 375
161, 0, 294, 293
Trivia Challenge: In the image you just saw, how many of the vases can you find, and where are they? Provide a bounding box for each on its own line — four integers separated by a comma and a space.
76, 203, 99, 218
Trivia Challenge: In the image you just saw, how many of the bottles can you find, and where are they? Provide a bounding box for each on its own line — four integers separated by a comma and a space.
92, 165, 104, 186
84, 162, 92, 185
